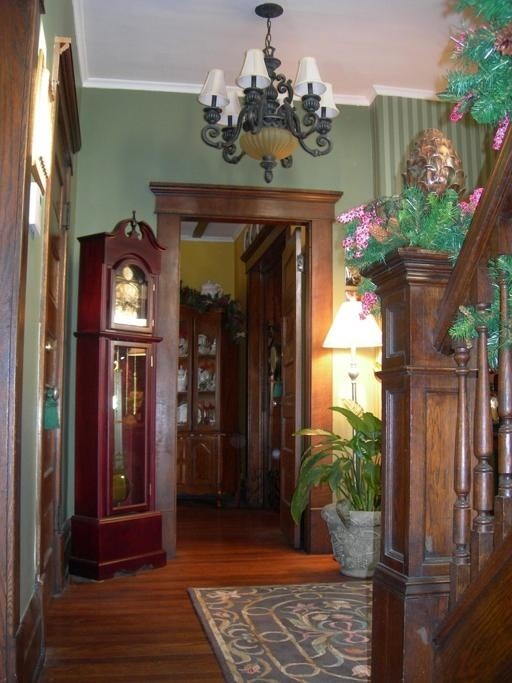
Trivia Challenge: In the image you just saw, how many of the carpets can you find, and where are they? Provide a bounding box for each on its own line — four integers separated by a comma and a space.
186, 580, 373, 682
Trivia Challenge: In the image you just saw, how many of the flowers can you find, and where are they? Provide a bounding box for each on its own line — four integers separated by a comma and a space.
435, 0, 512, 150
335, 185, 488, 320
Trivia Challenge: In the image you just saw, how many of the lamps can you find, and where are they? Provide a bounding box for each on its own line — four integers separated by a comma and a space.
197, 2, 341, 183
321, 300, 382, 492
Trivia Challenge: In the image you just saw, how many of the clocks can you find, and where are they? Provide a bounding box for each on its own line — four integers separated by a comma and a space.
70, 209, 167, 580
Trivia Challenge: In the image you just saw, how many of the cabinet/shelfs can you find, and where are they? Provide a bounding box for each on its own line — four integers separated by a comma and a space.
175, 279, 240, 511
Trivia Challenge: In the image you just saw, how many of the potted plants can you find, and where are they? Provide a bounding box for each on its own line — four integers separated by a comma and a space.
290, 397, 383, 579
